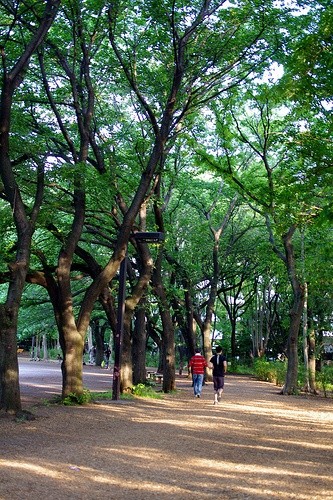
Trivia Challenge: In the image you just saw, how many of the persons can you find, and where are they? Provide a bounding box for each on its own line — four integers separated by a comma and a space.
186, 347, 209, 398
209, 346, 228, 405
82, 346, 112, 369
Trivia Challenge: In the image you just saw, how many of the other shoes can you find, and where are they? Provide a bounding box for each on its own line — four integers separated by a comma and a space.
218, 396, 222, 401
195, 394, 201, 398
214, 400, 218, 405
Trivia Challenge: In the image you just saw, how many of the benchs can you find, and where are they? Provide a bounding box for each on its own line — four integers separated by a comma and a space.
146, 370, 163, 384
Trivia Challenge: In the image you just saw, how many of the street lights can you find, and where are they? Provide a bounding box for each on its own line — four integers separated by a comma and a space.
112, 232, 165, 402
